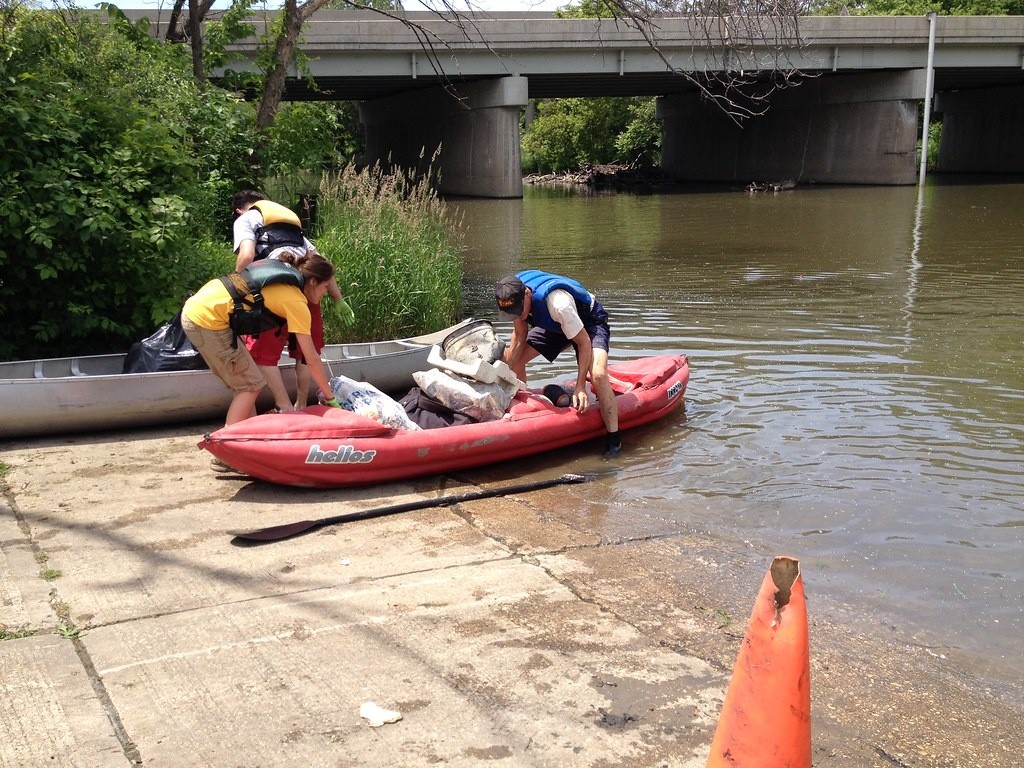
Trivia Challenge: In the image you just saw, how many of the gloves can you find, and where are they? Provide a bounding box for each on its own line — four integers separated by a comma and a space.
335, 300, 355, 326
325, 398, 341, 408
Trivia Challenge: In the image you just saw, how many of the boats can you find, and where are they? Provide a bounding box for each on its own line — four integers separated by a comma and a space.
0, 317, 476, 439
197, 353, 690, 489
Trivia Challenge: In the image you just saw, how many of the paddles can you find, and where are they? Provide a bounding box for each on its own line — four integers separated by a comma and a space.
225, 473, 586, 542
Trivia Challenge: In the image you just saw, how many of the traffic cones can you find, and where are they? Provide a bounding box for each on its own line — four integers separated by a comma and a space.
705, 555, 812, 768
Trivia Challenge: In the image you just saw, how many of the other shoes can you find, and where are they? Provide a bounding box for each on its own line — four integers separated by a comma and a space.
210, 458, 247, 474
605, 441, 623, 459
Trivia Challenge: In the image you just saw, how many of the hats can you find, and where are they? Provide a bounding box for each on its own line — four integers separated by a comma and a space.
495, 276, 525, 322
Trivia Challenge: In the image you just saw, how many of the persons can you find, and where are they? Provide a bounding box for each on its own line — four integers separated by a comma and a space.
232, 190, 355, 414
495, 270, 621, 454
181, 253, 342, 474
